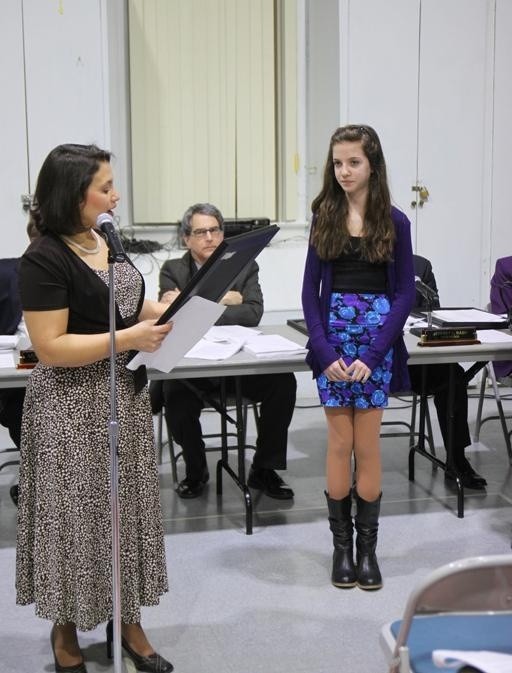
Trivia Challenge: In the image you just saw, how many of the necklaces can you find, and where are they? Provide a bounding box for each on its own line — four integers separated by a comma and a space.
60, 227, 102, 253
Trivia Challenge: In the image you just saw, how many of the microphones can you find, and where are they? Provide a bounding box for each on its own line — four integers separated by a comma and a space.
414, 275, 437, 302
96, 212, 125, 264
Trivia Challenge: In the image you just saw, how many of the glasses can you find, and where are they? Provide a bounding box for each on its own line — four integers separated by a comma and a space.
194, 226, 219, 237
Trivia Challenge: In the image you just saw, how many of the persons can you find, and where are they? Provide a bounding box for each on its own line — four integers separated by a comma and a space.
412, 254, 488, 489
21, 143, 173, 672
159, 204, 297, 500
301, 124, 415, 590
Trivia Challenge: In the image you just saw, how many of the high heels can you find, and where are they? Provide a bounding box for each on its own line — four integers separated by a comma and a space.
51, 623, 88, 673
106, 619, 173, 673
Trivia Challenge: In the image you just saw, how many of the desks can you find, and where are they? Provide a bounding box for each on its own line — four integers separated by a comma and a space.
0, 327, 311, 536
287, 312, 511, 518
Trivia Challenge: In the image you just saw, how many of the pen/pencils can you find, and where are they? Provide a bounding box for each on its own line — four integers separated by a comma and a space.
410, 318, 426, 325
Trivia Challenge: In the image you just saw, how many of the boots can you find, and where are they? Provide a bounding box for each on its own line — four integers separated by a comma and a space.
324, 488, 357, 587
353, 486, 383, 589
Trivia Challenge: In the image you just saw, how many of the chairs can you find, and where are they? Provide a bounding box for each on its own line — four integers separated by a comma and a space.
378, 390, 437, 468
474, 302, 511, 440
378, 555, 512, 673
157, 377, 259, 483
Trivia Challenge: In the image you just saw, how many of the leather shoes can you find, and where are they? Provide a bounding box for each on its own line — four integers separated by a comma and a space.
445, 459, 487, 488
178, 472, 209, 498
247, 464, 294, 500
